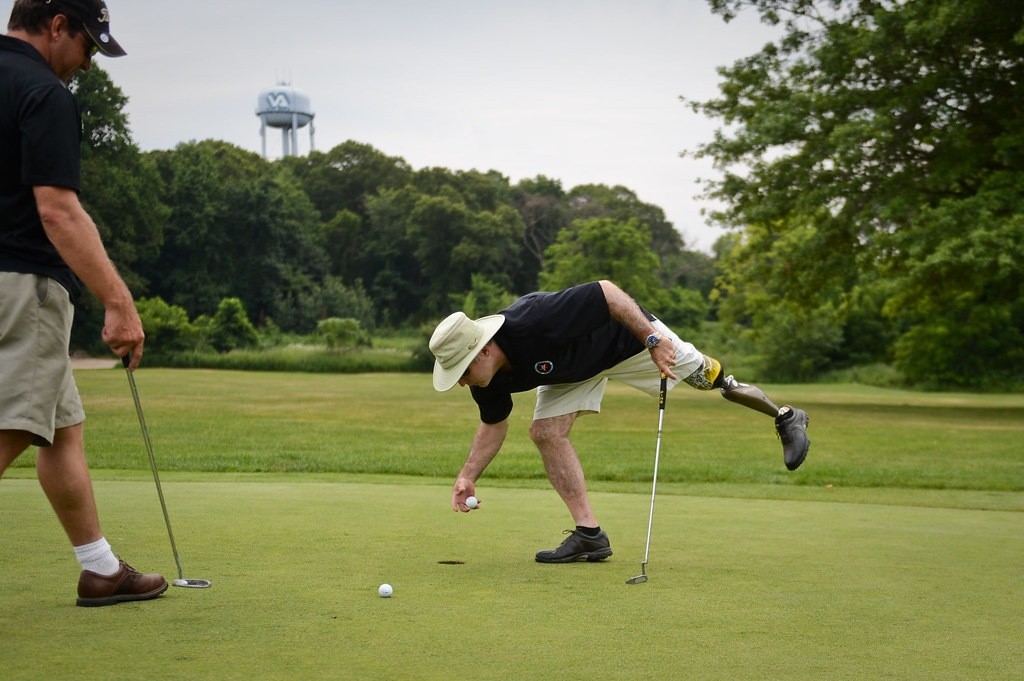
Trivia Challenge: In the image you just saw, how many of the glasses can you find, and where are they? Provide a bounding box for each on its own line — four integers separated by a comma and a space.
463, 367, 471, 376
79, 28, 99, 56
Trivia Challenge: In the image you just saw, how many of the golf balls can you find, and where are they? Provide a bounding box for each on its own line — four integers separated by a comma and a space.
466, 496, 478, 508
379, 584, 393, 598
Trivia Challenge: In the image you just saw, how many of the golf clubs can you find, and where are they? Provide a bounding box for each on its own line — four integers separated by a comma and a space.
120, 349, 212, 590
625, 338, 673, 585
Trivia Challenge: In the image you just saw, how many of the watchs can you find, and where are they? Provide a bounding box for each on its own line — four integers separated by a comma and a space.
644, 331, 661, 349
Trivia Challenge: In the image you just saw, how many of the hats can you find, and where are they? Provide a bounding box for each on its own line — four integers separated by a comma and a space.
428, 311, 505, 391
74, 0, 127, 58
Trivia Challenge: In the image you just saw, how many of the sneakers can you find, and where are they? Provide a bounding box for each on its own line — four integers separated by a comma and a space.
536, 531, 612, 563
775, 405, 810, 471
77, 559, 168, 606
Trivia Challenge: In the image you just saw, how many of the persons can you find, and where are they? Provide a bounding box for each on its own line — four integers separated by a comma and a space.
0, 0, 170, 609
427, 281, 810, 562
